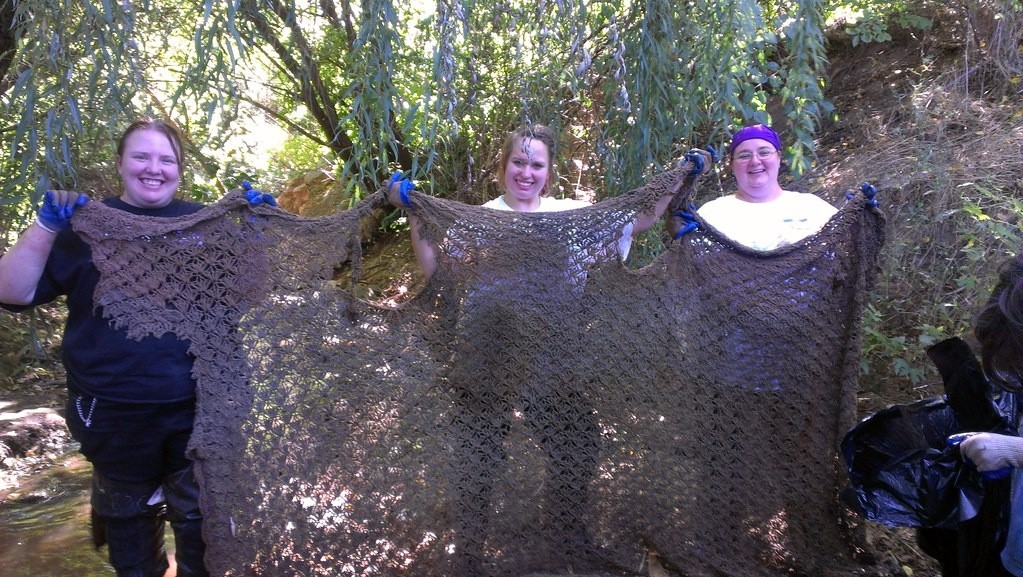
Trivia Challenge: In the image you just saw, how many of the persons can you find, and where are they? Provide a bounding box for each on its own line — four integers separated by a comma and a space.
667, 124, 880, 555
0, 122, 279, 576
948, 251, 1023, 577
384, 123, 718, 577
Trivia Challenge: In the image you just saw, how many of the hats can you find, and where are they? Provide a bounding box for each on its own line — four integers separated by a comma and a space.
728, 124, 781, 151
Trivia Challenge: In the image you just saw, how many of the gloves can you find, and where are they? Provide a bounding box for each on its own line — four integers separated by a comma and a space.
678, 145, 719, 178
665, 203, 699, 240
846, 181, 880, 207
240, 181, 277, 242
35, 189, 88, 235
386, 171, 416, 216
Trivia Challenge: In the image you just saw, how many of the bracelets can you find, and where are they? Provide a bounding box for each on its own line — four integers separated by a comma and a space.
33, 217, 57, 234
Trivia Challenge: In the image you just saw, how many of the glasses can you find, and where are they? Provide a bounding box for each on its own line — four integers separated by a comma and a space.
730, 151, 779, 163
